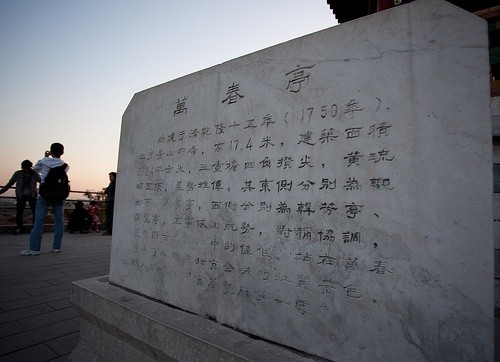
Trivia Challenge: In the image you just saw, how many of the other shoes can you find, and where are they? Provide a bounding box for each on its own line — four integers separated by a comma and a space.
89, 229, 93, 233
95, 229, 100, 233
51, 248, 61, 252
80, 230, 90, 234
16, 228, 22, 235
102, 232, 112, 235
20, 249, 41, 255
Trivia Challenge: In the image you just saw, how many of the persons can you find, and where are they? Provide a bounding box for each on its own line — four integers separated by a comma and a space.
21, 144, 70, 256
0, 160, 41, 235
67, 200, 100, 234
101, 172, 116, 236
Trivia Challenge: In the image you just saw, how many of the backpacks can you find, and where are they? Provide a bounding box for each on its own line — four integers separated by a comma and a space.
39, 163, 70, 207
